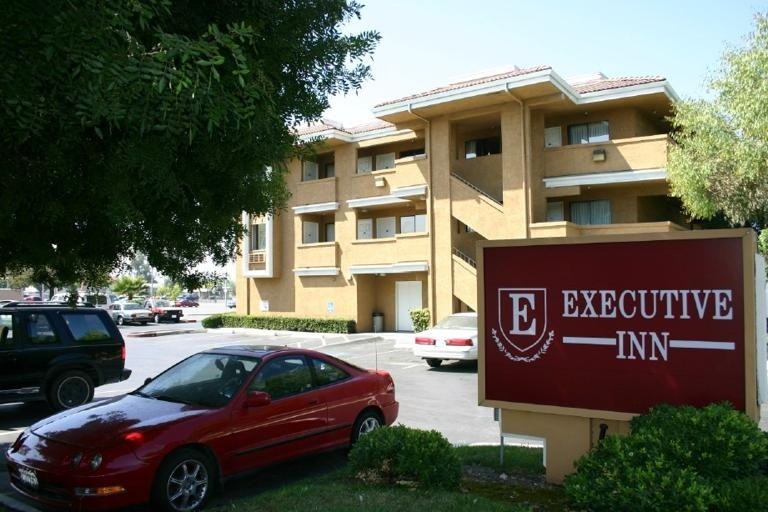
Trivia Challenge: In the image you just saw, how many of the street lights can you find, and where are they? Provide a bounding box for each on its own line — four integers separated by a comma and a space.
225, 272, 228, 300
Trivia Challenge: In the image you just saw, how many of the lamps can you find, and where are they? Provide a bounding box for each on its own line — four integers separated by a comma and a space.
374, 177, 386, 187
592, 148, 606, 162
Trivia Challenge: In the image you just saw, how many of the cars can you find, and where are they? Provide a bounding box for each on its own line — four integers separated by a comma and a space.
415, 313, 477, 367
176, 299, 199, 307
4, 345, 398, 512
228, 303, 235, 308
0, 300, 132, 412
24, 291, 184, 325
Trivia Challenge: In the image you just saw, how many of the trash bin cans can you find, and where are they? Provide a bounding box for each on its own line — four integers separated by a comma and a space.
373, 313, 384, 332
